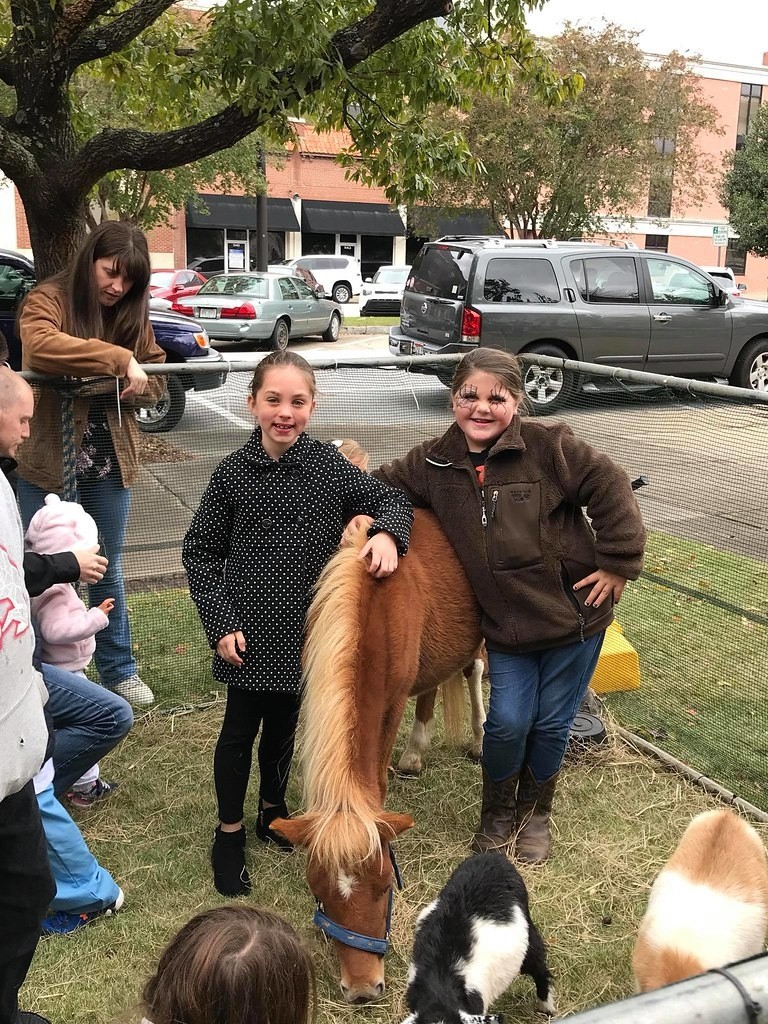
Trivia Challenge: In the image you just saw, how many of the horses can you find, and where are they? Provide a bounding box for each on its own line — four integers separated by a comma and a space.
265, 434, 490, 1010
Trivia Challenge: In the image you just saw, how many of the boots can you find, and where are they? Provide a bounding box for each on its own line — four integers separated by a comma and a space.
256, 798, 296, 852
471, 763, 520, 853
211, 822, 251, 896
515, 761, 562, 865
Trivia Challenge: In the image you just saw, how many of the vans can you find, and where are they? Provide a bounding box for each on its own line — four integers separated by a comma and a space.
697, 266, 734, 282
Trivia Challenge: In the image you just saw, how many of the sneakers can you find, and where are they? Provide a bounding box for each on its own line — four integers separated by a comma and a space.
67, 779, 119, 807
41, 886, 124, 935
115, 676, 154, 705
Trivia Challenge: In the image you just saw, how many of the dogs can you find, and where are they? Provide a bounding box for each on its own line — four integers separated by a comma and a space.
401, 853, 558, 1024
631, 807, 768, 996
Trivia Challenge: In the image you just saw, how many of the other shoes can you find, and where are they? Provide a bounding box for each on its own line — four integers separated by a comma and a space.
18, 1010, 51, 1024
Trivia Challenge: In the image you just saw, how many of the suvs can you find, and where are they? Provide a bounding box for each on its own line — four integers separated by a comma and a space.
388, 234, 768, 417
279, 255, 364, 304
1, 249, 229, 433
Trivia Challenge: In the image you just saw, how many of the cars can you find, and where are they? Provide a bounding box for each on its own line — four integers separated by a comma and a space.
712, 276, 747, 298
254, 264, 295, 275
170, 272, 343, 351
359, 264, 413, 316
147, 268, 207, 316
186, 256, 225, 279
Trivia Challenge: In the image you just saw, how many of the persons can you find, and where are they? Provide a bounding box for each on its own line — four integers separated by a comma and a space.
1, 331, 132, 794
11, 221, 165, 709
21, 491, 118, 810
1, 366, 127, 1024
338, 347, 648, 864
181, 350, 414, 898
142, 906, 316, 1023
